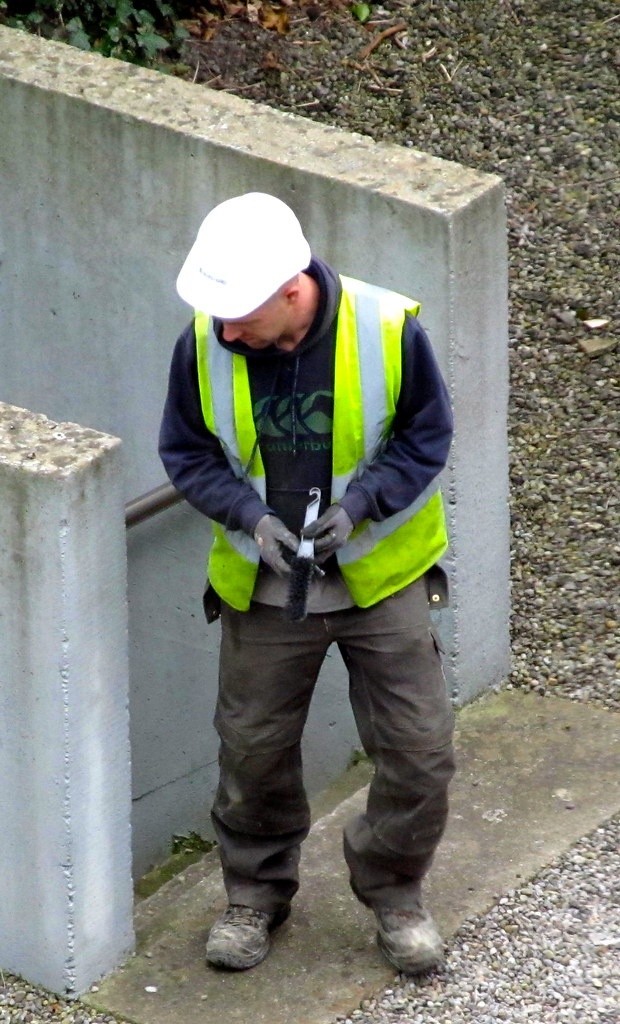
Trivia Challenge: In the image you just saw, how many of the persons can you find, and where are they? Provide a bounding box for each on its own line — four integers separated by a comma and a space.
159, 191, 458, 974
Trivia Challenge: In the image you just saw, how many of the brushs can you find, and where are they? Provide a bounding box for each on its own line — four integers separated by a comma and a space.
287, 487, 321, 623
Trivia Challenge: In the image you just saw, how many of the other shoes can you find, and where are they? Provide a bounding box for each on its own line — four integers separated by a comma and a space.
349, 874, 445, 976
204, 901, 290, 971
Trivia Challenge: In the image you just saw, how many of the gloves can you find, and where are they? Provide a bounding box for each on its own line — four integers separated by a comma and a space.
300, 503, 354, 564
253, 514, 300, 581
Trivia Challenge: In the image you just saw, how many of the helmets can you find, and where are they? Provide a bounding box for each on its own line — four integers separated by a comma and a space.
175, 192, 311, 320
